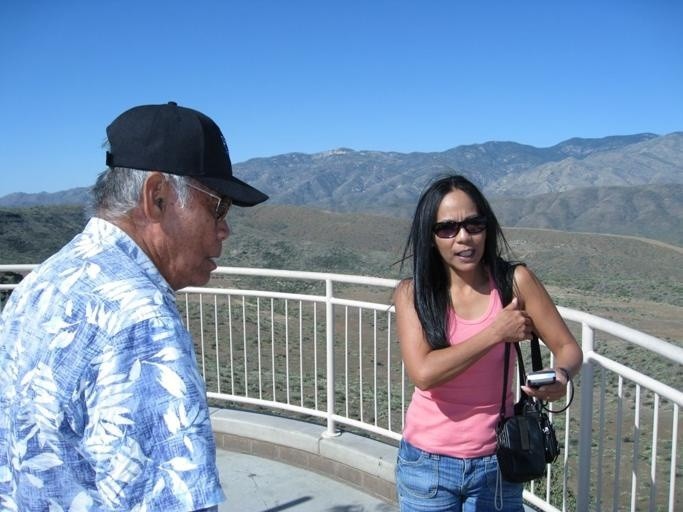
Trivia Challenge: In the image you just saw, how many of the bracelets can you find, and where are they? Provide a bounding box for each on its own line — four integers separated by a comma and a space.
559, 367, 570, 381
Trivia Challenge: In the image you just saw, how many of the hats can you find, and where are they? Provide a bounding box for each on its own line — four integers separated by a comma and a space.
100, 95, 270, 210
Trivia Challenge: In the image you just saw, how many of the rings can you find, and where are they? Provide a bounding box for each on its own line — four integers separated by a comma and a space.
545, 395, 550, 400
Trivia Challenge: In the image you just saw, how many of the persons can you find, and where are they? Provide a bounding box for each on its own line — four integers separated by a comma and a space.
387, 170, 584, 512
1, 99, 271, 512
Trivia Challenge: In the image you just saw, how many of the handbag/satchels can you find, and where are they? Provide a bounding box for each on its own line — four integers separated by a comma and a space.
496, 407, 547, 486
511, 393, 560, 465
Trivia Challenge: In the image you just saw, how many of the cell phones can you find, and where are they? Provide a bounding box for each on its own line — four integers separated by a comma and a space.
526, 370, 556, 387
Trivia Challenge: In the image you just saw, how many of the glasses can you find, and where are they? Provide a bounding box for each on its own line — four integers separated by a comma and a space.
167, 172, 234, 220
430, 213, 489, 240
